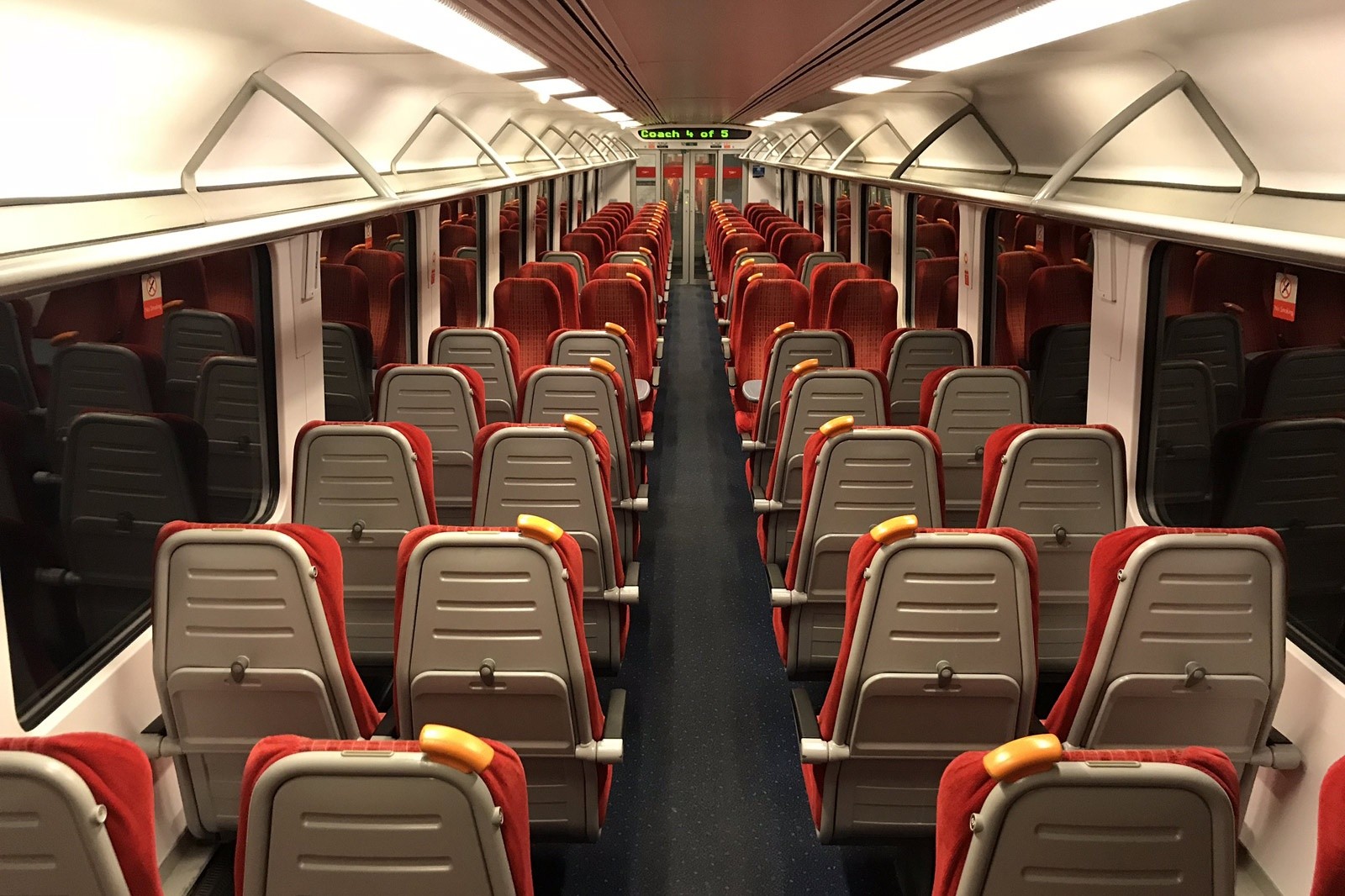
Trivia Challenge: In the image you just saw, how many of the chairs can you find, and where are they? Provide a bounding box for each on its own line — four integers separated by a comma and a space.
705, 202, 1345, 896
1, 202, 672, 895
